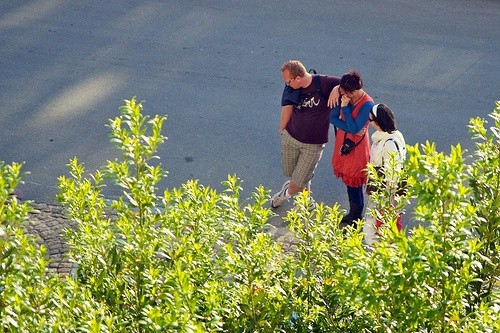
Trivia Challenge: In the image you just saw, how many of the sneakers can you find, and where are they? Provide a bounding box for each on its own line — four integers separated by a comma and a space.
305, 197, 316, 212
271, 180, 291, 209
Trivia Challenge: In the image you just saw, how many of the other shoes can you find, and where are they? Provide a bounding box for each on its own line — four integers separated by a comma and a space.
340, 214, 363, 223
342, 229, 364, 241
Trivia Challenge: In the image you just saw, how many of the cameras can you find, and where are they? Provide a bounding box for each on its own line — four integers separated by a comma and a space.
341, 138, 356, 155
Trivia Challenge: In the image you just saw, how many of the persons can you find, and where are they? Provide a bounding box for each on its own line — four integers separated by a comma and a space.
329, 71, 375, 231
271, 61, 342, 209
368, 103, 406, 246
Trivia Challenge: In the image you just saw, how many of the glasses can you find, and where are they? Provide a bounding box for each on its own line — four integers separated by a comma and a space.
368, 117, 375, 123
284, 74, 302, 87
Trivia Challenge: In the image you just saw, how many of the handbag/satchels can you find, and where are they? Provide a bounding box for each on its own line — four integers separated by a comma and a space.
366, 139, 408, 197
